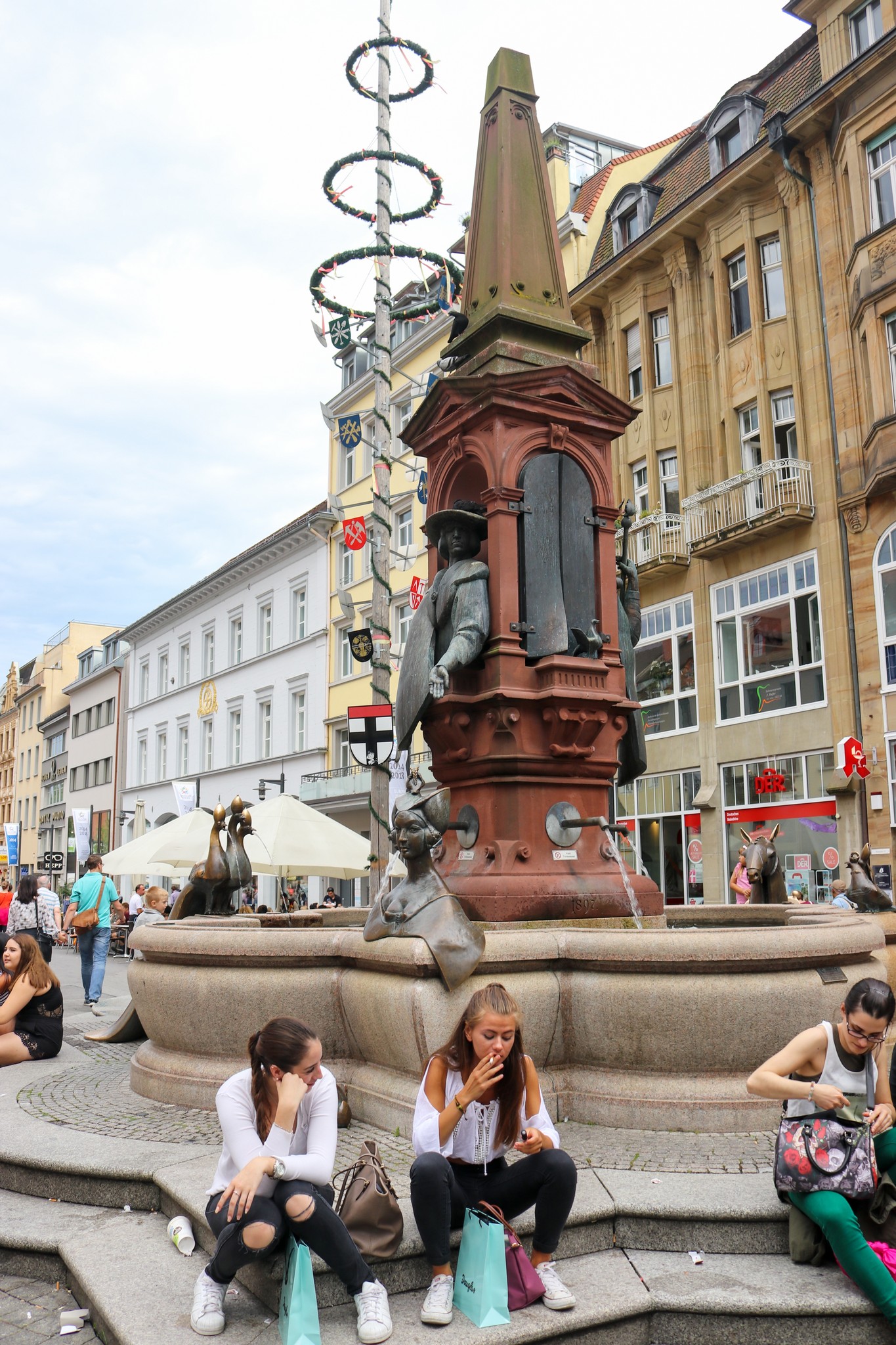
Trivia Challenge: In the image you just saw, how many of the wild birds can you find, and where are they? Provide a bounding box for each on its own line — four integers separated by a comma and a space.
85, 795, 256, 1042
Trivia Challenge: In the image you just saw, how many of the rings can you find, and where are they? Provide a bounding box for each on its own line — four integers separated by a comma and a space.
233, 1190, 240, 1198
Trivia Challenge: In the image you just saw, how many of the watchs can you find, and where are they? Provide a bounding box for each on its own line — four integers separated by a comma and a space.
268, 1157, 285, 1181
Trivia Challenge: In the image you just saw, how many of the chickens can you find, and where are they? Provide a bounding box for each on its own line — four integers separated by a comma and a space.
845, 843, 896, 913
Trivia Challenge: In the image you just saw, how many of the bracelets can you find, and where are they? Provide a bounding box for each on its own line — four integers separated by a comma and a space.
807, 1080, 816, 1102
454, 1094, 468, 1121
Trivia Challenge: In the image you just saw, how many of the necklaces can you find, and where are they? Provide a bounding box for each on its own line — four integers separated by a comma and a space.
265, 1086, 279, 1107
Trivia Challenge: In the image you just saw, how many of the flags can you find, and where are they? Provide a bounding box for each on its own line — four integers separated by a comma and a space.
3, 823, 20, 867
71, 806, 91, 861
170, 780, 196, 817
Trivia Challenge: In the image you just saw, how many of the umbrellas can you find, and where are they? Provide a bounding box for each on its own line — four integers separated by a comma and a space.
86, 806, 221, 892
148, 793, 370, 912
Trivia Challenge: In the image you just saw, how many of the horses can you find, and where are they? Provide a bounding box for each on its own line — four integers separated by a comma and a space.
738, 823, 788, 905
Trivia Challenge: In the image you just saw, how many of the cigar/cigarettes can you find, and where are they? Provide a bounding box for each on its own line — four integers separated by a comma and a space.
490, 1055, 495, 1064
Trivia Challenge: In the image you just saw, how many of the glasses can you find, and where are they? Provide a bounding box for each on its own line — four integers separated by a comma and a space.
845, 1011, 890, 1043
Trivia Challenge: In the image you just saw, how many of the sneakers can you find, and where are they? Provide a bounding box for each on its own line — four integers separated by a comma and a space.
83, 999, 97, 1006
351, 1278, 395, 1345
417, 1272, 455, 1324
536, 1263, 573, 1312
187, 1262, 230, 1335
110, 945, 124, 954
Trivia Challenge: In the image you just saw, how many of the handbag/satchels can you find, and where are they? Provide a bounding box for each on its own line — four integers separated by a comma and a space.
772, 1109, 877, 1200
35, 932, 53, 963
70, 908, 99, 932
454, 1207, 510, 1327
478, 1198, 548, 1314
278, 1231, 323, 1345
334, 1141, 405, 1258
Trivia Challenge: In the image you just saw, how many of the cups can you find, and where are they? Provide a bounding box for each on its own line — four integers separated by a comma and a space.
167, 1215, 196, 1254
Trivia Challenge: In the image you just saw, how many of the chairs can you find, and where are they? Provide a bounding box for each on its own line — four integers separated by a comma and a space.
60, 911, 136, 959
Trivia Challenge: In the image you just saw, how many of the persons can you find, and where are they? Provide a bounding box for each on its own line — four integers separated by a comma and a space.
830, 879, 855, 910
417, 501, 491, 698
616, 558, 651, 787
727, 844, 752, 904
56, 853, 125, 1017
0, 874, 346, 965
747, 977, 896, 1331
360, 766, 485, 992
791, 890, 816, 906
0, 933, 64, 1068
0, 932, 17, 1006
189, 1014, 394, 1345
408, 982, 577, 1326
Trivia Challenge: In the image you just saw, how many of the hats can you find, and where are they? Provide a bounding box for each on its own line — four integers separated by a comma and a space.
830, 879, 846, 892
